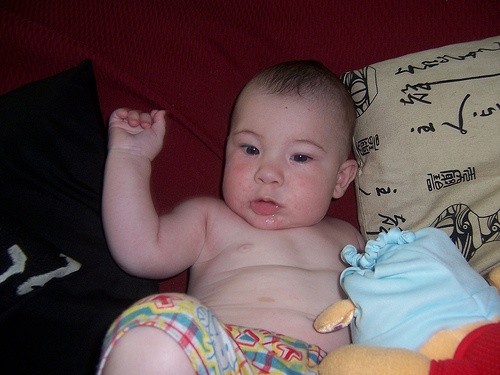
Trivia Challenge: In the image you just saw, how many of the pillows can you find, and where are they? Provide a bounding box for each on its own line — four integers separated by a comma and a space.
340, 36, 500, 277
0, 58, 161, 375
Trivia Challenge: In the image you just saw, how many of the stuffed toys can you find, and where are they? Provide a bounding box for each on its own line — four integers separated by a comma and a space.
312, 226, 500, 375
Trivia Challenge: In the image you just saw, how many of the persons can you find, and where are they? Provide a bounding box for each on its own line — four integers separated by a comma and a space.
96, 61, 367, 375
